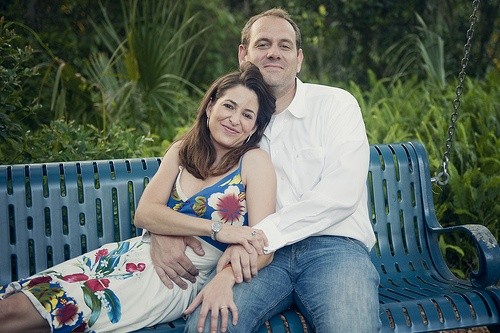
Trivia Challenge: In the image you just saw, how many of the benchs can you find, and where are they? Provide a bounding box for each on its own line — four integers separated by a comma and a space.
0, 139, 500, 333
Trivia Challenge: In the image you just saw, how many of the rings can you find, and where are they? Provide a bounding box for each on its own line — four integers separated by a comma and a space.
220, 306, 230, 309
252, 231, 256, 236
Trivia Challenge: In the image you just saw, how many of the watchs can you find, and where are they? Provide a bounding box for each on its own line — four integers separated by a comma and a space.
211, 221, 224, 240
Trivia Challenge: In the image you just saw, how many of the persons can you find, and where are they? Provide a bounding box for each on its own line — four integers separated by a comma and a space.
133, 8, 383, 333
0, 61, 278, 333
